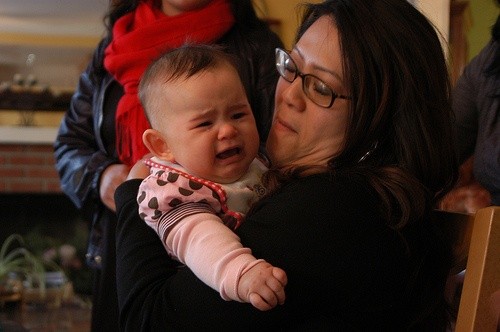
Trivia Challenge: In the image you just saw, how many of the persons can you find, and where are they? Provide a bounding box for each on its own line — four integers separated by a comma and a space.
53, 0, 285, 332
113, 0, 459, 332
137, 45, 287, 312
438, 14, 499, 213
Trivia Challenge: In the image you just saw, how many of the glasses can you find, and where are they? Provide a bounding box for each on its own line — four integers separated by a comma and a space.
274, 47, 351, 108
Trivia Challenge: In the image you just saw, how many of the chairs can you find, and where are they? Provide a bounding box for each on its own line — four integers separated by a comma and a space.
433, 205, 500, 332
